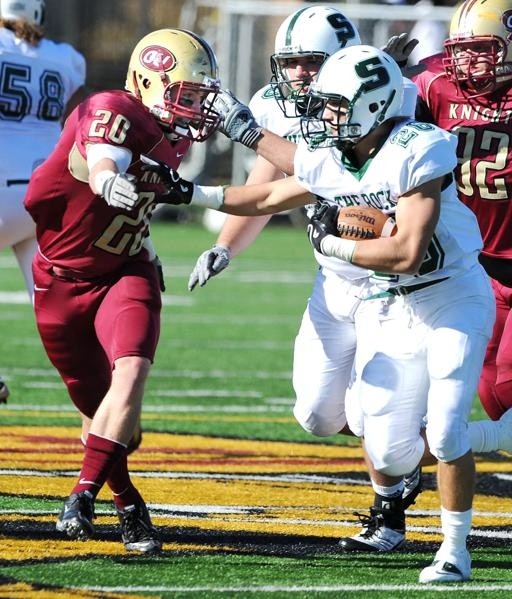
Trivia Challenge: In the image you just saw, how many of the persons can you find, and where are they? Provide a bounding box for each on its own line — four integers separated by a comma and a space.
379, 0, 512, 423
1, 1, 88, 405
22, 27, 221, 556
186, 4, 424, 552
138, 42, 497, 583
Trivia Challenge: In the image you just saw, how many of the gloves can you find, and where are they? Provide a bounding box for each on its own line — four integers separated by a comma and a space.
99, 172, 138, 212
305, 199, 339, 256
380, 31, 420, 71
204, 86, 263, 151
138, 153, 196, 206
186, 244, 232, 291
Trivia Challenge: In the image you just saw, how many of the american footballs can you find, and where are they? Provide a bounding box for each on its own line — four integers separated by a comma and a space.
330, 206, 396, 243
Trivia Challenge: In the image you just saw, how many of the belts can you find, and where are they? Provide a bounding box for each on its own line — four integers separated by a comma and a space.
385, 276, 451, 297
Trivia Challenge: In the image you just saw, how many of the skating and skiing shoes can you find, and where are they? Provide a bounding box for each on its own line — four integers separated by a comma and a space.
417, 549, 474, 584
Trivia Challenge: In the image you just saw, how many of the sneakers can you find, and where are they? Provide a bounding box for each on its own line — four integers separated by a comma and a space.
403, 463, 421, 513
54, 488, 97, 541
335, 508, 407, 553
114, 500, 162, 553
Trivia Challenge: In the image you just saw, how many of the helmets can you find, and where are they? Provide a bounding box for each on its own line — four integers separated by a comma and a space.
298, 42, 405, 152
440, 0, 512, 102
123, 26, 225, 144
267, 5, 363, 121
0, 0, 49, 27
